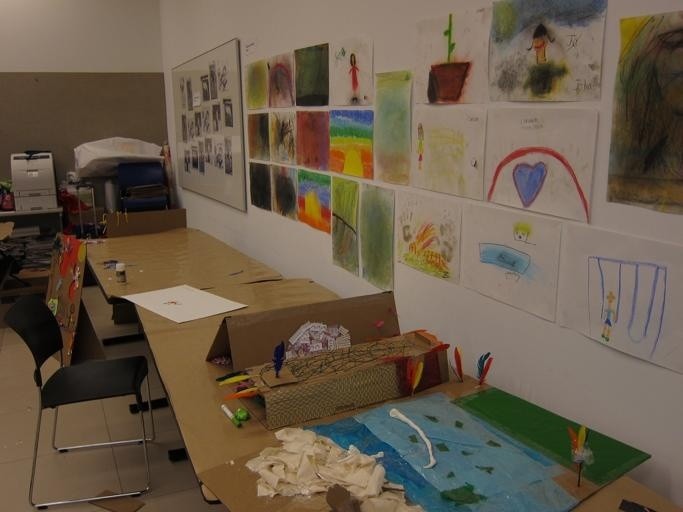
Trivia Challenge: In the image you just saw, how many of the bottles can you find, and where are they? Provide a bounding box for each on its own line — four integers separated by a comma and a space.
116, 263, 126, 283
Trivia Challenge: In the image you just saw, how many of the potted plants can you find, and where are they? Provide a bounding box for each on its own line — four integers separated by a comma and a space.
1, 179, 15, 211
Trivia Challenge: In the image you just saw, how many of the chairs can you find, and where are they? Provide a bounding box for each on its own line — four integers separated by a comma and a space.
6, 295, 156, 508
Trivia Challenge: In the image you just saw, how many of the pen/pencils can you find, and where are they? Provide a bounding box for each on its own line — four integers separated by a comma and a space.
221, 403, 241, 428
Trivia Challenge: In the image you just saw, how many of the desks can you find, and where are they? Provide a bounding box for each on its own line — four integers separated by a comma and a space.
569, 474, 683, 512
0, 207, 65, 234
135, 277, 492, 504
80, 227, 284, 415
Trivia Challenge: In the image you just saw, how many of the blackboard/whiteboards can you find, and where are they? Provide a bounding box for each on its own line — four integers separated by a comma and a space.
169, 37, 247, 213
